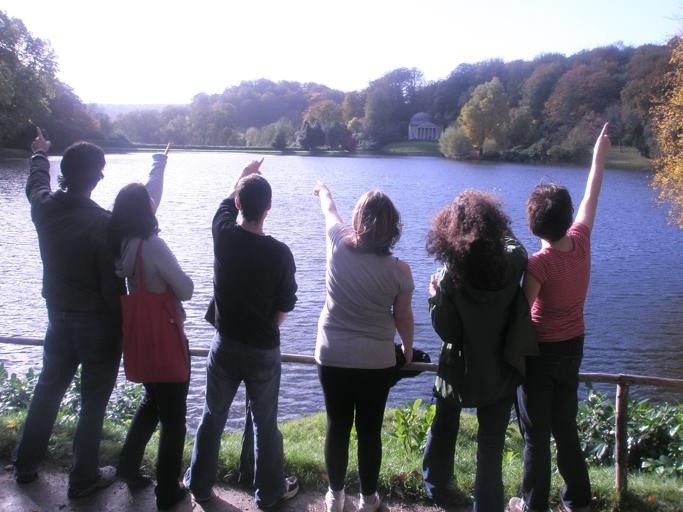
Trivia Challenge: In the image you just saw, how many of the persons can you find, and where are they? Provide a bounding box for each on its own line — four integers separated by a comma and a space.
14, 125, 122, 497
183, 158, 300, 510
421, 190, 540, 512
509, 121, 612, 512
313, 178, 415, 511
112, 140, 187, 511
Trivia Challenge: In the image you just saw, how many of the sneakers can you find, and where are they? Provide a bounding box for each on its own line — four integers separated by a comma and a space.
256, 476, 300, 508
183, 467, 212, 502
324, 486, 345, 511
359, 491, 379, 512
156, 484, 186, 509
506, 496, 549, 511
122, 470, 151, 491
68, 465, 118, 498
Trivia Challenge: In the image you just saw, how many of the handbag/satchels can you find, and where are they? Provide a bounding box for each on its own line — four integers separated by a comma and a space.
119, 238, 191, 385
